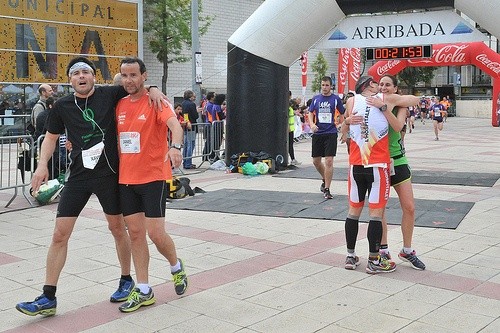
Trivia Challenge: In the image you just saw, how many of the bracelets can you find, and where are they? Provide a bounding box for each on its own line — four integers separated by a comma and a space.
344, 116, 350, 125
149, 85, 159, 91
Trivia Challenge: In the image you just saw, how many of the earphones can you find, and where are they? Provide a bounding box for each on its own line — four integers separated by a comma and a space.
94, 79, 95, 83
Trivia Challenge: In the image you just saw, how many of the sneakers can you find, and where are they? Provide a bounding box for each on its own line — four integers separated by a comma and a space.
320, 179, 325, 192
119, 287, 156, 313
171, 258, 188, 295
380, 250, 392, 260
110, 279, 136, 302
345, 256, 359, 270
366, 254, 396, 274
398, 249, 426, 271
324, 188, 333, 198
15, 294, 57, 316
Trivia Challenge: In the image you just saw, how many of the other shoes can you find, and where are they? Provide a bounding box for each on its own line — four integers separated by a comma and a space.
190, 166, 196, 169
291, 159, 302, 166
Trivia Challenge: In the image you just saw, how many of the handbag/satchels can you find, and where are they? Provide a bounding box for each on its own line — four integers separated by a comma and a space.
31, 174, 65, 206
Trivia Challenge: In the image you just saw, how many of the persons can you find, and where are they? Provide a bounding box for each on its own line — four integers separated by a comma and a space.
290, 97, 316, 141
201, 91, 226, 161
15, 56, 170, 315
343, 92, 355, 155
428, 96, 447, 141
308, 75, 346, 199
65, 57, 188, 312
30, 84, 54, 130
427, 96, 451, 123
407, 105, 415, 134
344, 73, 427, 273
35, 98, 60, 180
181, 90, 203, 169
288, 90, 302, 165
172, 103, 192, 174
418, 95, 428, 126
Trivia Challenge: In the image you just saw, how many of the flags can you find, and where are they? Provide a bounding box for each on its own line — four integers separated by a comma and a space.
348, 48, 361, 93
338, 48, 350, 98
302, 51, 308, 95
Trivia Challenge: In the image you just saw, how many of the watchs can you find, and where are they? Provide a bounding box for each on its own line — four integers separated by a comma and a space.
171, 143, 181, 152
379, 104, 387, 111
